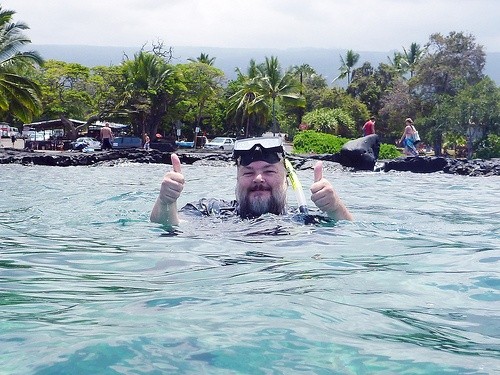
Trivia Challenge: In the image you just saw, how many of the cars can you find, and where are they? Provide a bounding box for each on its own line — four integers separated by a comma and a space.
1, 118, 248, 157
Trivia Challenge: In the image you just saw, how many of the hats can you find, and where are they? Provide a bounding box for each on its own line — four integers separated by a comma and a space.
237, 150, 283, 167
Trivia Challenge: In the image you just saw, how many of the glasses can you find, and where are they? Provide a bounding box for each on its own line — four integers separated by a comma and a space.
234, 138, 285, 159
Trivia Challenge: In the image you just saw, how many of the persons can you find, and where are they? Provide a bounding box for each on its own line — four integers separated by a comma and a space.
11, 122, 150, 151
363, 116, 375, 136
150, 135, 353, 225
399, 118, 417, 156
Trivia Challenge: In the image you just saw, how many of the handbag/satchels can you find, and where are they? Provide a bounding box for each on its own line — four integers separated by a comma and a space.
410, 125, 420, 144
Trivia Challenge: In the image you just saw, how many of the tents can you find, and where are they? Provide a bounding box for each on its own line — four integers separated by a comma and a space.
23, 118, 127, 141
